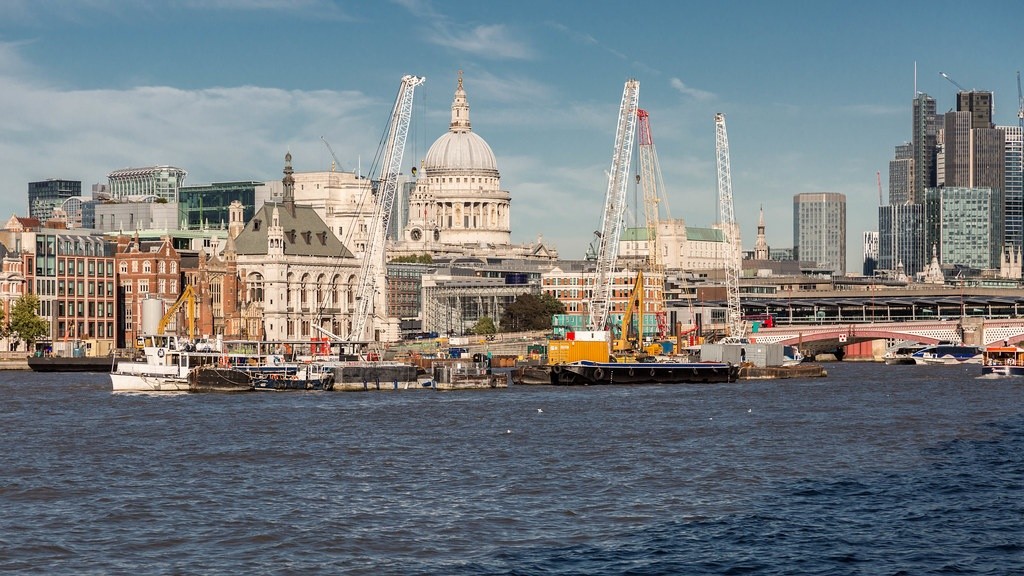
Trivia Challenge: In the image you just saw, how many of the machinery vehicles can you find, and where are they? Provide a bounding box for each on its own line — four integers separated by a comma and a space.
130, 284, 198, 361
547, 267, 663, 362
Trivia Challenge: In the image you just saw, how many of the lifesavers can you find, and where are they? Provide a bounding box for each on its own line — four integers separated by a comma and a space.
158, 349, 164, 359
274, 356, 280, 366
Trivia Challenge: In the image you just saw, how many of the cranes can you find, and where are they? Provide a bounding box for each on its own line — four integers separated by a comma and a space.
587, 79, 642, 334
711, 113, 744, 343
633, 109, 699, 339
309, 74, 428, 359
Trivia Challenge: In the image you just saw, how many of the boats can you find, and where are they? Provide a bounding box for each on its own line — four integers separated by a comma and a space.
25, 352, 131, 372
542, 349, 741, 384
980, 345, 1023, 377
108, 334, 335, 397
